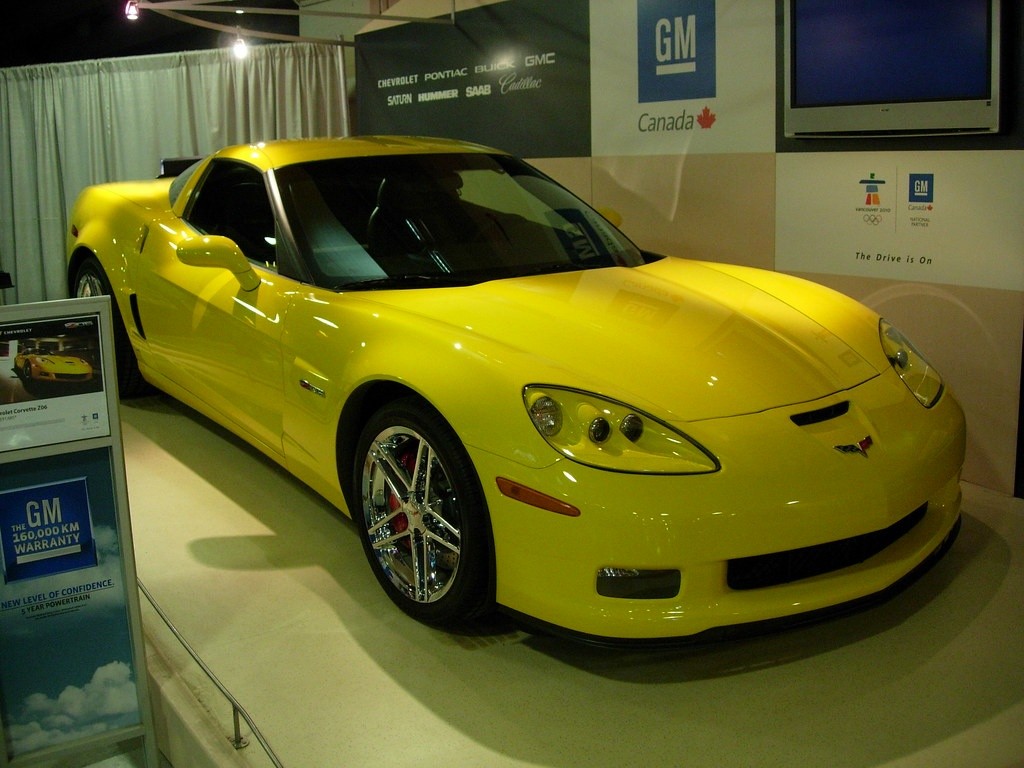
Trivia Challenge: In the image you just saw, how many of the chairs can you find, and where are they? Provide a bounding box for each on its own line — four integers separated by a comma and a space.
368, 170, 519, 274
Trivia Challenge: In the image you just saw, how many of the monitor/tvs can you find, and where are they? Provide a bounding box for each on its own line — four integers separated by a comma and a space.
783, 0, 1002, 142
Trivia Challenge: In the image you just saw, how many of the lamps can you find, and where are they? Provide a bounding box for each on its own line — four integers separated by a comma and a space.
125, 0, 140, 19
232, 25, 249, 59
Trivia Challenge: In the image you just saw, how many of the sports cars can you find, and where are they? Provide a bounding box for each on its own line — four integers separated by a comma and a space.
63, 134, 970, 650
12, 336, 92, 390
